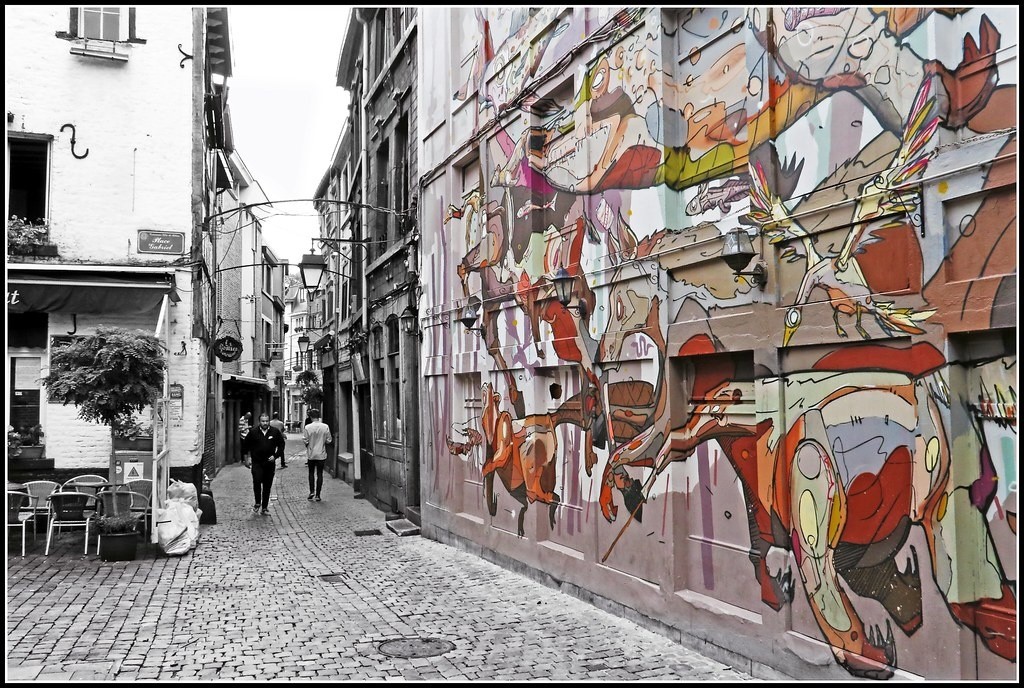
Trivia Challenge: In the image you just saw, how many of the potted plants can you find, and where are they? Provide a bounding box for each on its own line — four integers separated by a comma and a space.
137, 423, 153, 451
93, 514, 140, 561
8, 214, 58, 257
8, 424, 45, 459
301, 387, 324, 409
114, 413, 138, 450
295, 370, 319, 386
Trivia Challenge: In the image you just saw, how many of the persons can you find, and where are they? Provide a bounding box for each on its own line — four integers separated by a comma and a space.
238, 412, 252, 468
241, 412, 285, 517
301, 409, 333, 501
302, 409, 312, 467
269, 412, 288, 469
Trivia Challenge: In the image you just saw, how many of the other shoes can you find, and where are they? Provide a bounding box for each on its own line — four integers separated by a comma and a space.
307, 494, 314, 499
316, 498, 321, 501
253, 504, 260, 513
261, 509, 272, 516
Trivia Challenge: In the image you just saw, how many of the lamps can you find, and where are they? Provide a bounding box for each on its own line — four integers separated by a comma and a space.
294, 326, 325, 353
717, 226, 768, 285
460, 305, 487, 340
550, 261, 588, 320
400, 306, 423, 344
298, 237, 372, 302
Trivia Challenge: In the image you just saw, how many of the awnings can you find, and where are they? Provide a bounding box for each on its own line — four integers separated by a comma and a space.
7, 264, 182, 316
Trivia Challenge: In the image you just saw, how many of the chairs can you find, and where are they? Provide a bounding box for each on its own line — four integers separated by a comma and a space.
44, 492, 99, 555
20, 480, 62, 540
8, 491, 39, 556
115, 479, 152, 547
96, 490, 148, 556
58, 474, 107, 504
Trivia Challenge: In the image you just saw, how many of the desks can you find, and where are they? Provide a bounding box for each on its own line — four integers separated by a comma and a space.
64, 482, 126, 512
8, 483, 27, 491
